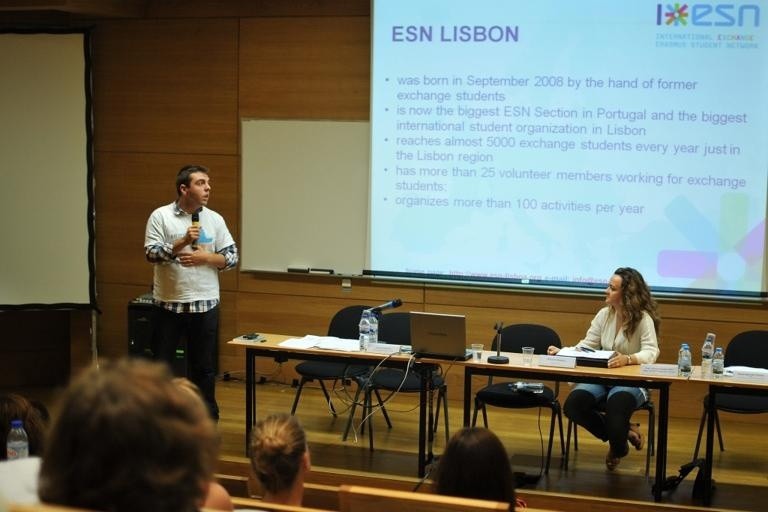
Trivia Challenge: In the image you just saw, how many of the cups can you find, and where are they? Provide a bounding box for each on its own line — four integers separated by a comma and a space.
521, 346, 535, 368
471, 343, 484, 364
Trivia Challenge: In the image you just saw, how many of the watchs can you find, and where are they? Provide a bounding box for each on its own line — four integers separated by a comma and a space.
625, 354, 632, 365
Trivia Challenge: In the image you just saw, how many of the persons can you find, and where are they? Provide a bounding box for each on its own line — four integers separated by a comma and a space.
547, 266, 661, 469
0, 393, 46, 461
435, 427, 527, 507
36, 357, 234, 512
247, 413, 312, 509
143, 164, 238, 432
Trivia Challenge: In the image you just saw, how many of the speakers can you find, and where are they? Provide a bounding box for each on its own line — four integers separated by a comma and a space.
127, 292, 187, 385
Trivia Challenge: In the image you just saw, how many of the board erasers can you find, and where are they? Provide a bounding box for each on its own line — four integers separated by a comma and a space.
286, 264, 309, 274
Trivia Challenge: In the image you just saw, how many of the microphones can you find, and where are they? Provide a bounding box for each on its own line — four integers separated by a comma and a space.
191, 213, 199, 250
492, 319, 504, 334
368, 298, 403, 314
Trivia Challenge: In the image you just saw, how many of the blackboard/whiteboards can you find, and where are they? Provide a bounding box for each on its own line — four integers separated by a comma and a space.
238, 117, 368, 279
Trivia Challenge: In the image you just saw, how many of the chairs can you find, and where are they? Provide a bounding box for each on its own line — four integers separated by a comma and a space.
561, 403, 655, 478
692, 330, 768, 460
472, 325, 564, 476
291, 305, 381, 436
341, 311, 450, 446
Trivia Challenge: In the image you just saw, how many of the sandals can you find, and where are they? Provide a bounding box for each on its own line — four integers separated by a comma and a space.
605, 444, 622, 471
629, 422, 645, 450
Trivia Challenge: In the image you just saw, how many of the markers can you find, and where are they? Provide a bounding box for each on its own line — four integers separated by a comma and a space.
309, 271, 330, 275
311, 268, 334, 274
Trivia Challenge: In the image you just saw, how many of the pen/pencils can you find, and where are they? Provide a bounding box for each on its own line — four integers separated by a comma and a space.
579, 345, 595, 355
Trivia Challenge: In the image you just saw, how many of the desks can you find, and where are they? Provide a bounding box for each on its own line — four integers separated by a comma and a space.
226, 332, 768, 478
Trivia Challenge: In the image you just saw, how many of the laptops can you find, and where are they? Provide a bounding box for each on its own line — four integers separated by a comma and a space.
410, 311, 473, 361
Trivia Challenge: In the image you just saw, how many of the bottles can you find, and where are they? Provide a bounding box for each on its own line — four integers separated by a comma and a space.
678, 344, 692, 376
712, 348, 724, 374
360, 311, 370, 348
7, 421, 29, 463
369, 313, 378, 349
702, 337, 713, 373
